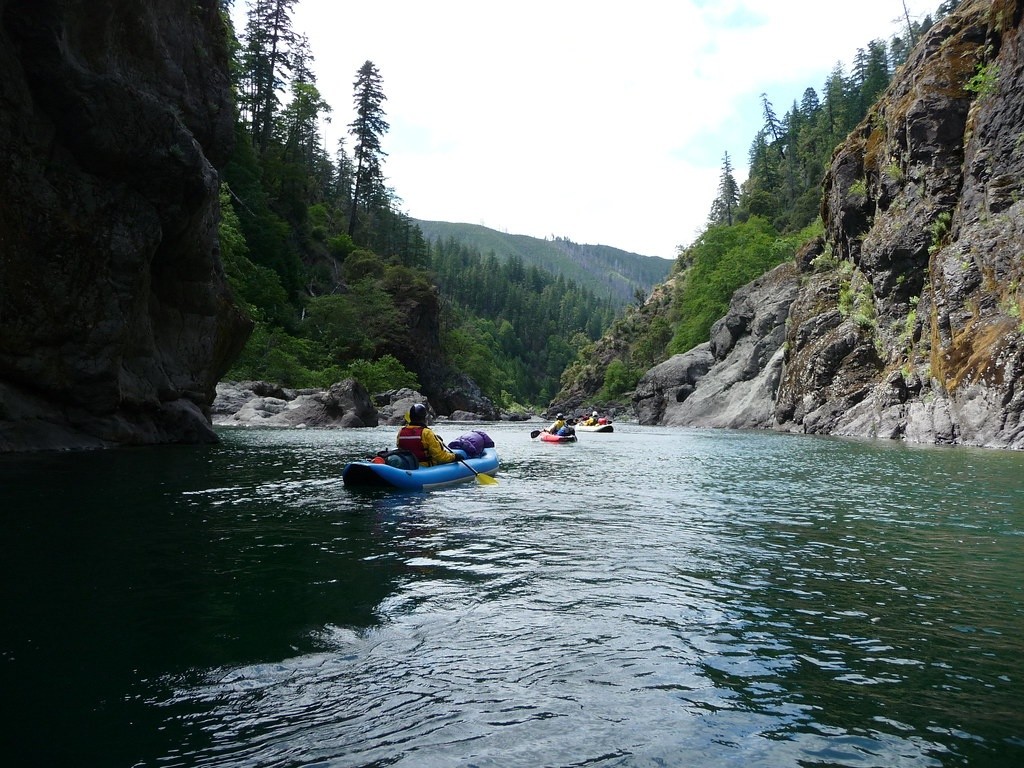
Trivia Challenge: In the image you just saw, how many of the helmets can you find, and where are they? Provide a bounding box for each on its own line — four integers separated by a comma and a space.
410, 404, 427, 422
592, 411, 598, 416
556, 413, 563, 419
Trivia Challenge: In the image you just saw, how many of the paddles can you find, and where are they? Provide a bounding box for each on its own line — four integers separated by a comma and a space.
531, 430, 544, 439
434, 436, 500, 484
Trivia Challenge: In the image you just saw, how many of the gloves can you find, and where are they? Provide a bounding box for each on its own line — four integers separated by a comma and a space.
455, 453, 465, 461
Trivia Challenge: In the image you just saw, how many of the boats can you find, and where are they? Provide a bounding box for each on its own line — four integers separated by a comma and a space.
541, 427, 578, 442
574, 422, 614, 432
343, 447, 500, 493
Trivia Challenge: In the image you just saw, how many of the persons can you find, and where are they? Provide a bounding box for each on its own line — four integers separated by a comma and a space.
546, 414, 567, 435
574, 411, 600, 427
397, 405, 465, 469
